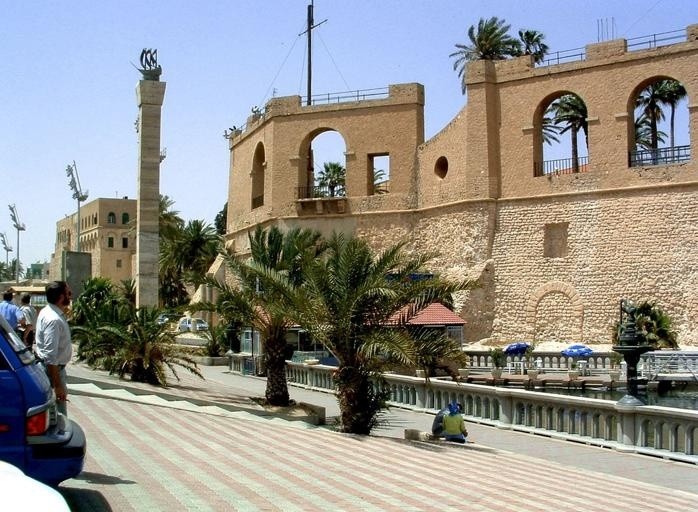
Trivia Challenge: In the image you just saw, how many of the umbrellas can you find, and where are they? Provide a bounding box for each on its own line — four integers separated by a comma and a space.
502, 341, 532, 361
560, 344, 594, 368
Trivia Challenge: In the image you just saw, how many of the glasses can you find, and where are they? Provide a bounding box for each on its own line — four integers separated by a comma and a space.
68, 291, 71, 296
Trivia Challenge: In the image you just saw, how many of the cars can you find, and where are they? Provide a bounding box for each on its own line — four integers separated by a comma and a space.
178, 317, 208, 333
0, 313, 87, 490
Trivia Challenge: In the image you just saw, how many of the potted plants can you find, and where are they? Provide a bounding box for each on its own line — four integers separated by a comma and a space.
451, 351, 471, 376
415, 359, 427, 378
524, 348, 539, 377
568, 364, 579, 381
489, 347, 505, 380
607, 352, 623, 381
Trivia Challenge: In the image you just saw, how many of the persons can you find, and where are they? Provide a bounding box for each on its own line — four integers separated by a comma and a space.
431, 403, 463, 437
0, 289, 26, 335
14, 292, 38, 354
442, 401, 475, 444
34, 280, 73, 419
